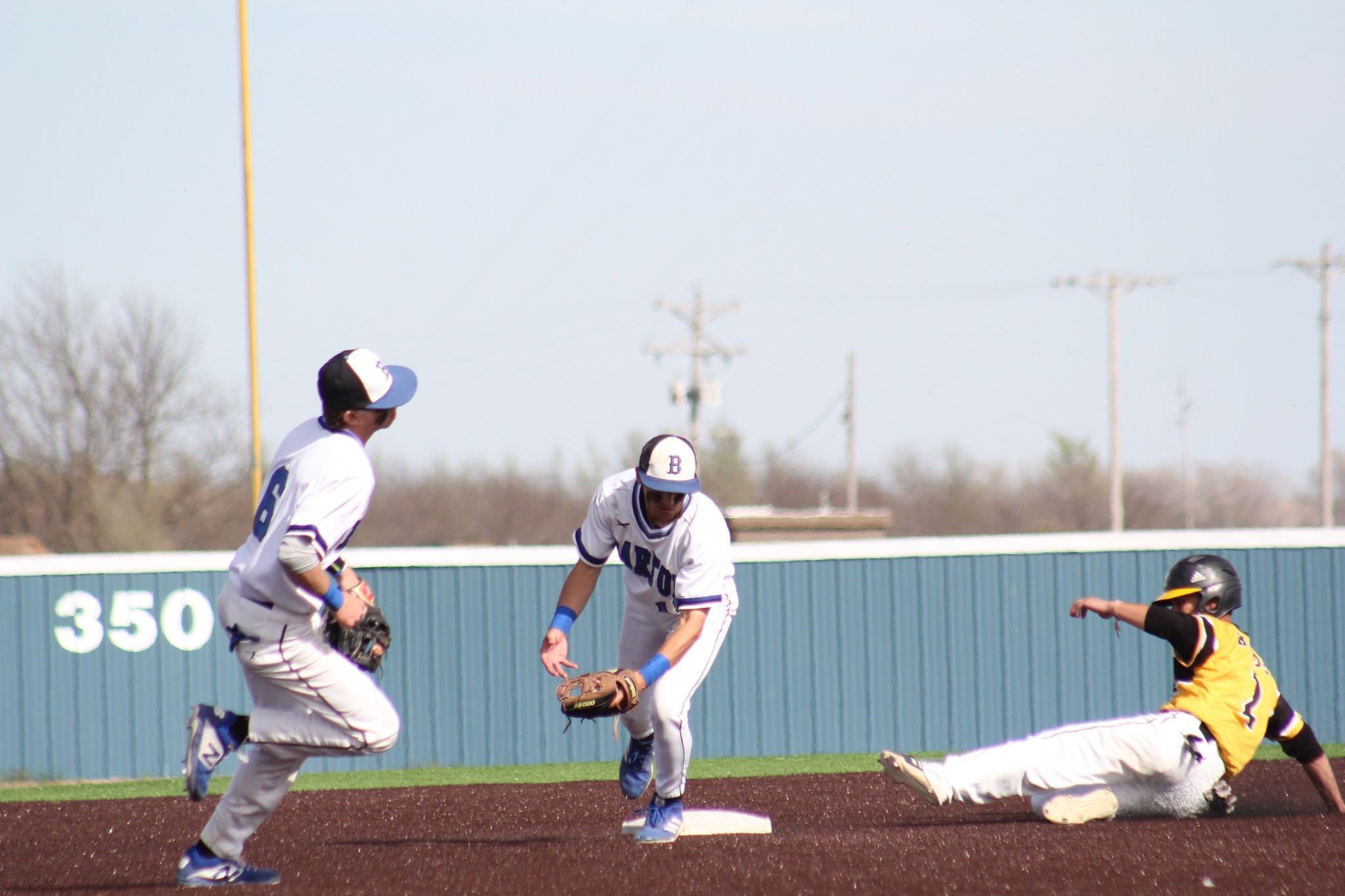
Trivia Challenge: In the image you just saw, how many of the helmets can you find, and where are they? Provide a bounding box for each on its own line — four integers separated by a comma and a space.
1150, 554, 1243, 619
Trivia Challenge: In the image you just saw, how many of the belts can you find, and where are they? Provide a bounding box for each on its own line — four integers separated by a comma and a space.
1199, 722, 1215, 744
248, 597, 273, 609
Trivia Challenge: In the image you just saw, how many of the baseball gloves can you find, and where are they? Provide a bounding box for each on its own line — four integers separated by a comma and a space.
328, 581, 392, 675
556, 670, 643, 720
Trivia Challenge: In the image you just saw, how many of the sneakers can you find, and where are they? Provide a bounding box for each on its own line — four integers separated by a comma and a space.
175, 845, 281, 888
619, 731, 655, 800
877, 750, 941, 807
633, 792, 684, 844
181, 703, 241, 803
1041, 788, 1120, 825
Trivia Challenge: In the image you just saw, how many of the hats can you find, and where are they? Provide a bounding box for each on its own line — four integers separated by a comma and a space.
318, 348, 418, 410
638, 434, 702, 494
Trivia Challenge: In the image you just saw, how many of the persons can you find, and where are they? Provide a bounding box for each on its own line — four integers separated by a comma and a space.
879, 555, 1345, 826
539, 434, 739, 844
175, 348, 418, 886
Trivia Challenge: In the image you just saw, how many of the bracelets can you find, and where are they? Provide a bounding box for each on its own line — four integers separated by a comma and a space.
1114, 601, 1123, 620
